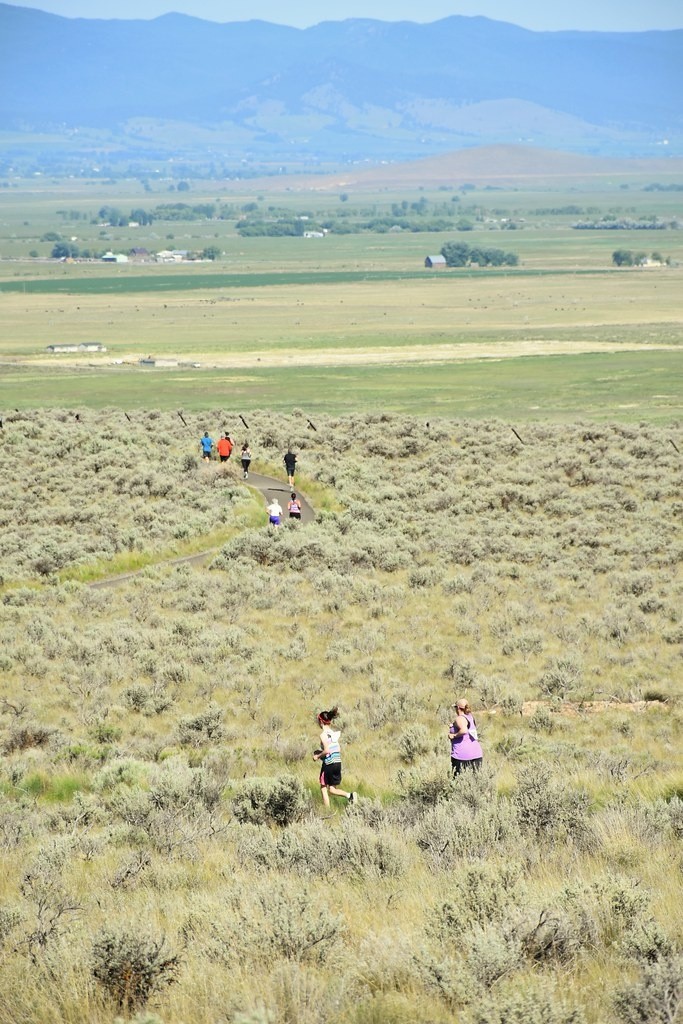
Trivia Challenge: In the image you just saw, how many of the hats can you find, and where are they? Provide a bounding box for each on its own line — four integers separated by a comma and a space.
451, 698, 468, 709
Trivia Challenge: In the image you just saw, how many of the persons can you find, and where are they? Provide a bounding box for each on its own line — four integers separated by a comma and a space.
225, 432, 235, 446
240, 444, 251, 479
312, 707, 357, 808
217, 434, 232, 463
288, 493, 301, 520
200, 432, 214, 462
284, 448, 297, 492
448, 699, 483, 777
266, 498, 283, 525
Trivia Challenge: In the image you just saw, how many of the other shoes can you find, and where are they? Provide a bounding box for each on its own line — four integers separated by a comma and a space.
347, 791, 357, 805
243, 476, 247, 480
290, 485, 294, 492
